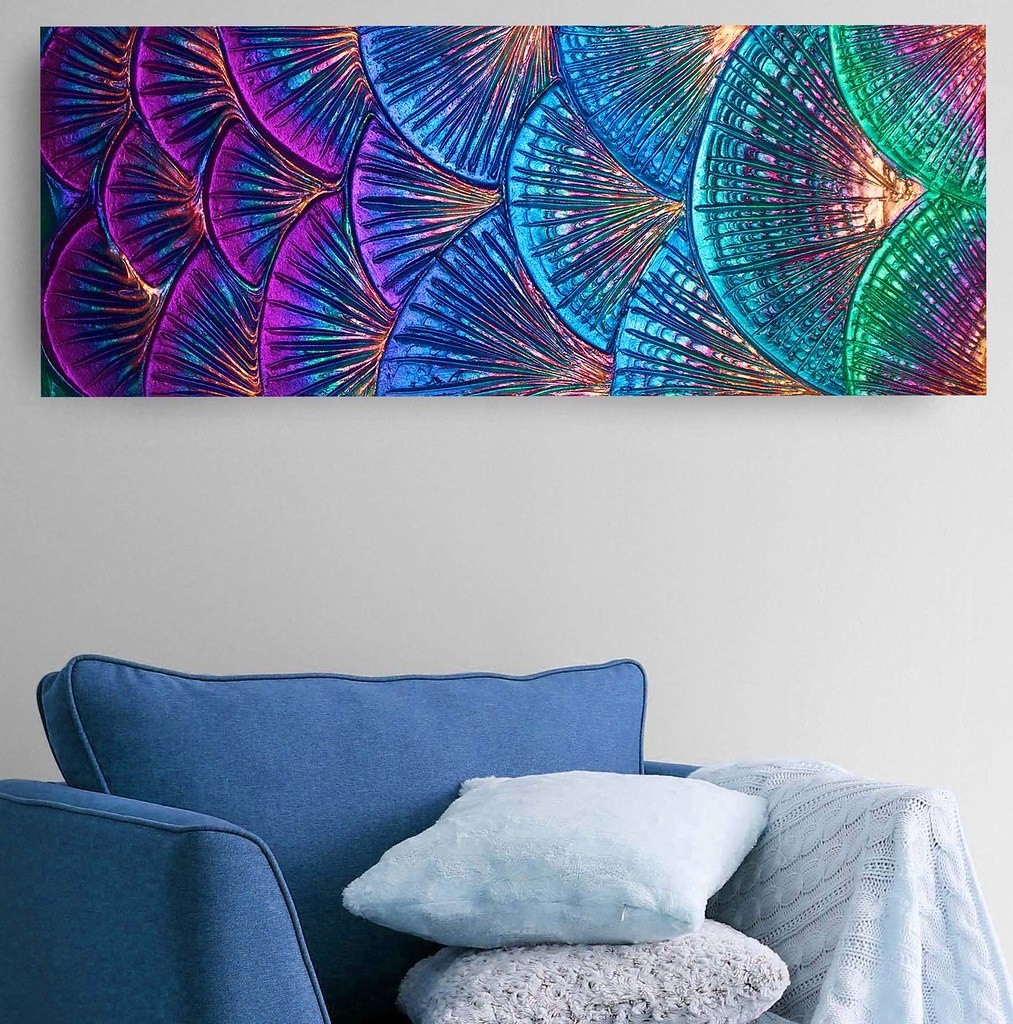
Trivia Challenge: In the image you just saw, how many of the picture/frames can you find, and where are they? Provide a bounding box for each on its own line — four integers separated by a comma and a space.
41, 25, 985, 398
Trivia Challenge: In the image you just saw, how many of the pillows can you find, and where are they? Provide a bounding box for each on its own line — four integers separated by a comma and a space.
343, 770, 767, 950
395, 919, 790, 1024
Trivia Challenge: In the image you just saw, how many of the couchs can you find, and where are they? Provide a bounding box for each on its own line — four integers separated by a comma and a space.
0, 655, 1013, 1024
